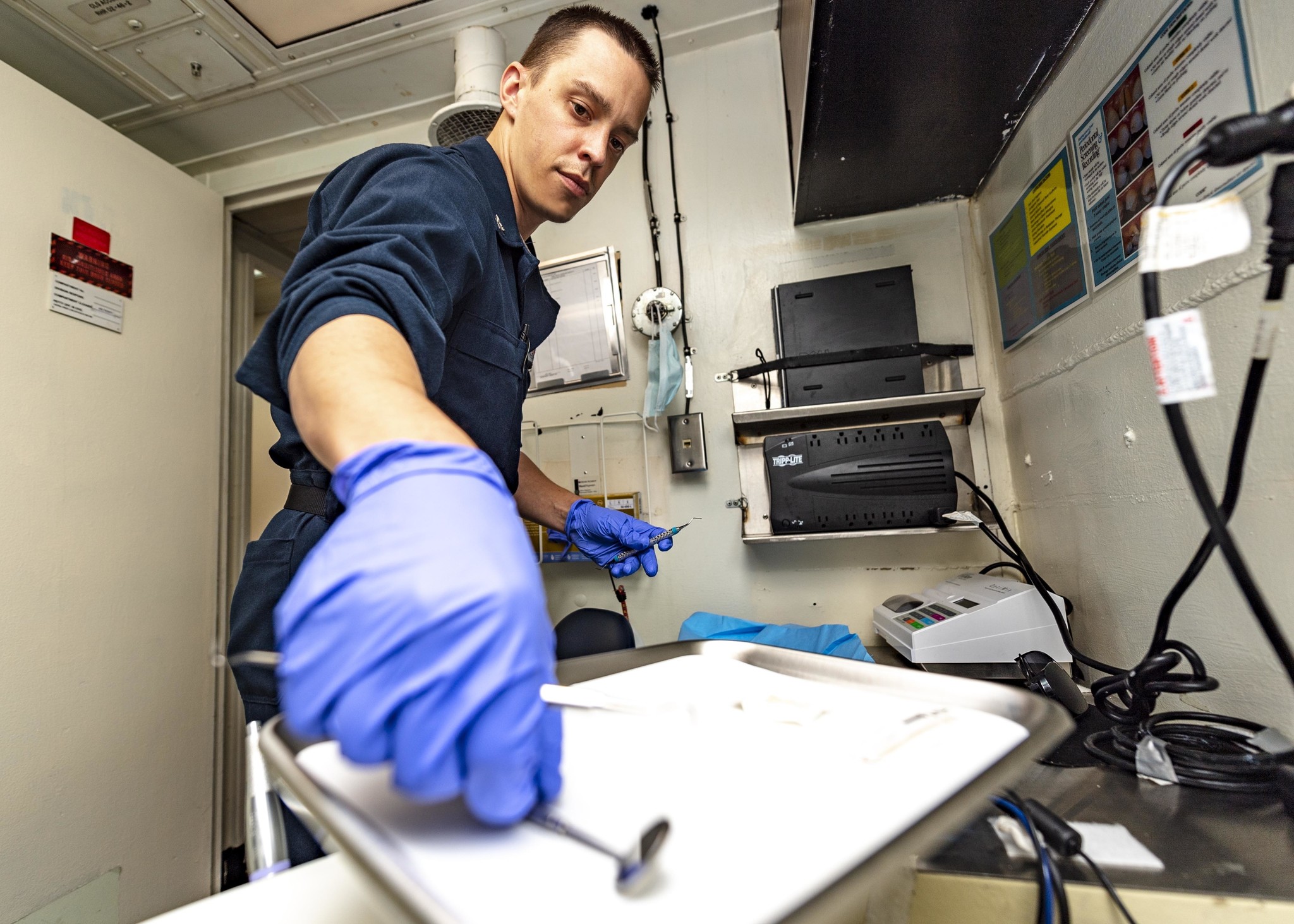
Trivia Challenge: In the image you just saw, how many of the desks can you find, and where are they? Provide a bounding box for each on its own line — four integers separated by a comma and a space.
864, 644, 1294, 924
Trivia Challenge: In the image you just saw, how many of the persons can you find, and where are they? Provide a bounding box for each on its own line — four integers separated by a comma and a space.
218, 4, 674, 876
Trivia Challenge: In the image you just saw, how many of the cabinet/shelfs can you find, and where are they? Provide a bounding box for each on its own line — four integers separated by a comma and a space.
729, 387, 1001, 544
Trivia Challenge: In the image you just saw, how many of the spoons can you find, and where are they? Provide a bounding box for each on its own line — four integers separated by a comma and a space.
524, 814, 670, 885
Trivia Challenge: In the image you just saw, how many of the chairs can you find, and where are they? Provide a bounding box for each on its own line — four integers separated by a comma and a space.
552, 608, 636, 660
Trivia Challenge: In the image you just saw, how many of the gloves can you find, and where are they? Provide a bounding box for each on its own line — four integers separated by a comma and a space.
565, 499, 675, 577
272, 442, 565, 827
